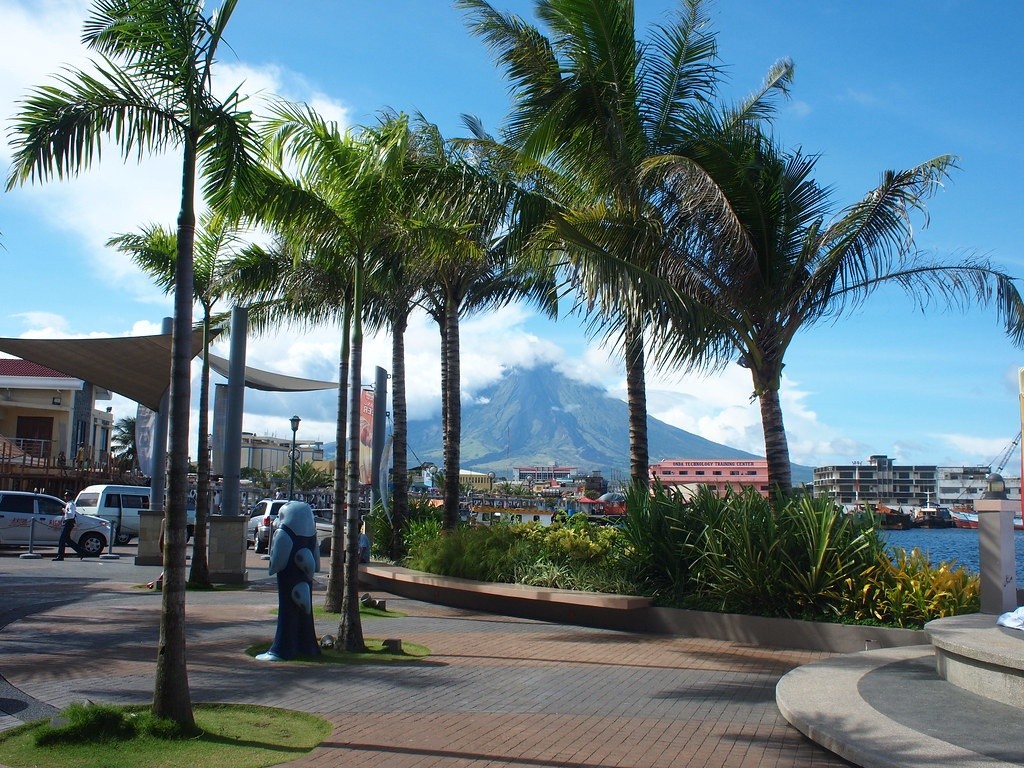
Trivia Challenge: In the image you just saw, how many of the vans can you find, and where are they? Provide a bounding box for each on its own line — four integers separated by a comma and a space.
74, 485, 195, 546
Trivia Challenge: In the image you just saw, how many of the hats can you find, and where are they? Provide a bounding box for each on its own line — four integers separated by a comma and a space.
64, 488, 76, 496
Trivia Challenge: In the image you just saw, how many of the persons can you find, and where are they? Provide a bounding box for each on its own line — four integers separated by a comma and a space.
51, 490, 86, 562
357, 519, 371, 564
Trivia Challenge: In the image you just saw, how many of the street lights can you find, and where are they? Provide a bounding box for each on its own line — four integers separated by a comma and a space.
429, 465, 437, 497
487, 471, 496, 492
526, 473, 533, 489
290, 415, 302, 501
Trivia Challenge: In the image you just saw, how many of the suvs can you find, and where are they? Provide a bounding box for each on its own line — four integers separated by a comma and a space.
0, 491, 115, 557
247, 498, 300, 553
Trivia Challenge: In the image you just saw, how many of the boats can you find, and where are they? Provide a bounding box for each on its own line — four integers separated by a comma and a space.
946, 431, 1023, 531
915, 488, 943, 528
868, 502, 914, 530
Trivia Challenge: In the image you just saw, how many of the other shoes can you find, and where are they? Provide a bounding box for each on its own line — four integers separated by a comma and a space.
52, 555, 64, 561
